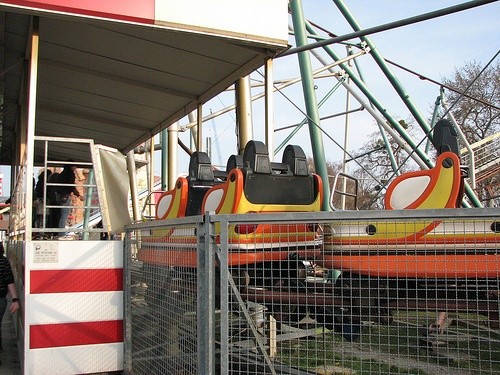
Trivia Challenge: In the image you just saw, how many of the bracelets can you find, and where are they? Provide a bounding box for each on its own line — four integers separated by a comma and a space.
12, 298, 19, 302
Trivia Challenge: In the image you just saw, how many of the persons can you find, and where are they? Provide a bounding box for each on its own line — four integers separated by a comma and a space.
0, 242, 20, 366
33, 159, 85, 240
